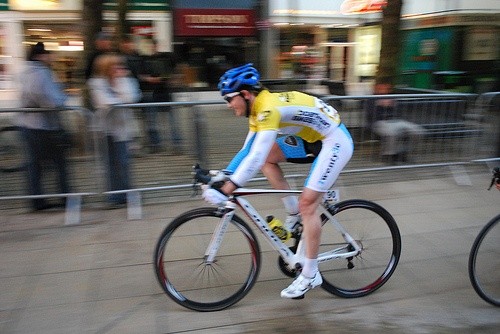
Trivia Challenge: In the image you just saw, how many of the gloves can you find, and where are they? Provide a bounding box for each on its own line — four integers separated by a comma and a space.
209, 172, 231, 185
201, 188, 234, 206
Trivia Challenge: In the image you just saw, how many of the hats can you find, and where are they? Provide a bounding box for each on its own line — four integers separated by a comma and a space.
31, 42, 51, 54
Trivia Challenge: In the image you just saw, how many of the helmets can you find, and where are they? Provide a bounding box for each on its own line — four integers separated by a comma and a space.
217, 62, 262, 96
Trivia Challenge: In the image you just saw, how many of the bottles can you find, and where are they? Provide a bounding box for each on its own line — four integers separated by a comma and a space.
267, 215, 292, 243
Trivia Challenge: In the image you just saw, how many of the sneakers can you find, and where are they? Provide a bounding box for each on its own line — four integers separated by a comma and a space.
285, 213, 302, 232
280, 271, 323, 299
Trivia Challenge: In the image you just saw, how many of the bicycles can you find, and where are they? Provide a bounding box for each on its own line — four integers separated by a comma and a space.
468, 167, 500, 307
152, 164, 401, 313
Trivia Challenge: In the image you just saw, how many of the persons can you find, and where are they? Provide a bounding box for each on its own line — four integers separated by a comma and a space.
199, 63, 354, 298
17, 33, 178, 210
363, 83, 436, 166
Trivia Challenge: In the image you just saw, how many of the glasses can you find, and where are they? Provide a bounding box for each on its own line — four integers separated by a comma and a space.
223, 92, 241, 103
